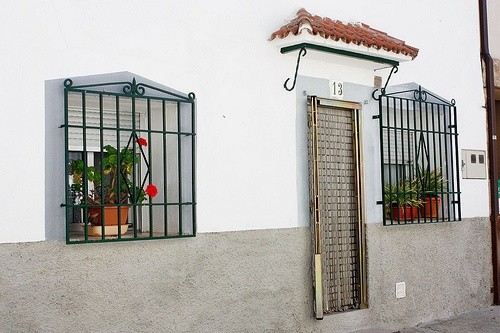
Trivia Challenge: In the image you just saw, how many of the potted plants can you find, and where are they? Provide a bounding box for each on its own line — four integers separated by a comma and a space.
383, 181, 423, 221
412, 165, 449, 217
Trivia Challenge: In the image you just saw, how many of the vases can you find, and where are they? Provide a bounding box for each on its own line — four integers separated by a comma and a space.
86, 206, 130, 238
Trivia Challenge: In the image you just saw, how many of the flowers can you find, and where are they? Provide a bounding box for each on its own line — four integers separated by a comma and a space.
67, 137, 158, 207
